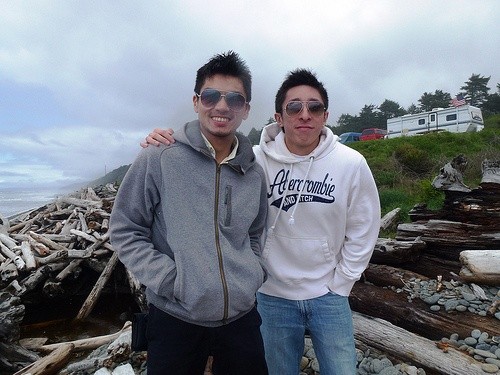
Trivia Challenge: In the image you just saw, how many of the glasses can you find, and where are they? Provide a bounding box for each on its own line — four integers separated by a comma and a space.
281, 100, 325, 118
195, 89, 249, 113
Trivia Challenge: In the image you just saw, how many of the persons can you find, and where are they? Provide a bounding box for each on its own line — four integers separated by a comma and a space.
139, 69, 381, 375
108, 48, 271, 375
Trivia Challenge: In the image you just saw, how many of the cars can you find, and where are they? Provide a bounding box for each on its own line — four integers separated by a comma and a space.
360, 128, 387, 142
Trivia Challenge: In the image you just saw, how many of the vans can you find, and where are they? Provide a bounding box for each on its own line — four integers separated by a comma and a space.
338, 132, 363, 143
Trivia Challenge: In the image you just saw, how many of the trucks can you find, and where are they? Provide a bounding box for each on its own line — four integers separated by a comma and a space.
387, 103, 484, 139
